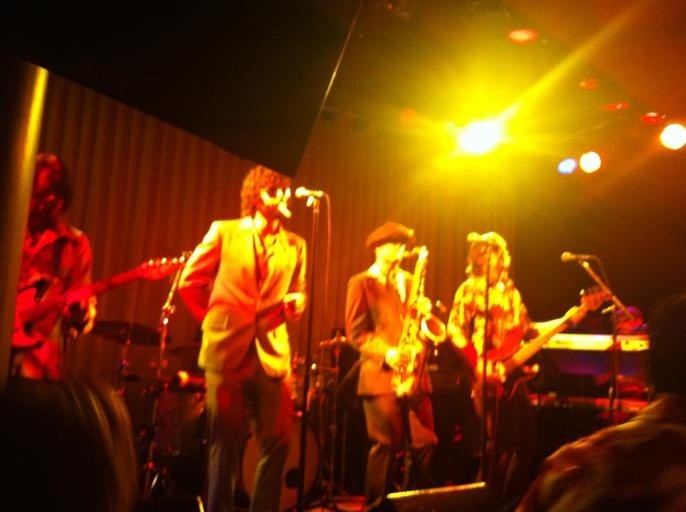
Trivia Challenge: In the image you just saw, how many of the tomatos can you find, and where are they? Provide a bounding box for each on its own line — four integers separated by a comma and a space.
523, 392, 651, 420
521, 330, 649, 353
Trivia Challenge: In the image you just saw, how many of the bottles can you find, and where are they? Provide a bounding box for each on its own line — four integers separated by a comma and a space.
468, 230, 511, 268
366, 221, 413, 246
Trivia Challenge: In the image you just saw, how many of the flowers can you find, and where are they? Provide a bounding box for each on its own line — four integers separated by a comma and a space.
466, 230, 493, 247
295, 187, 326, 198
560, 249, 598, 265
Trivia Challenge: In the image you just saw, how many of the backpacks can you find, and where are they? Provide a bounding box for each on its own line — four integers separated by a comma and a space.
173, 396, 319, 512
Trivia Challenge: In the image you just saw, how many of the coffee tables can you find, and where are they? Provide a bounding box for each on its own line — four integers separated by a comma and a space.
83, 316, 170, 347
167, 343, 203, 360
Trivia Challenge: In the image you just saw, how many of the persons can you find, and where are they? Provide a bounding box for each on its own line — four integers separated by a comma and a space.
176, 165, 308, 512
446, 232, 542, 482
515, 286, 686, 512
9, 151, 97, 381
344, 221, 439, 512
